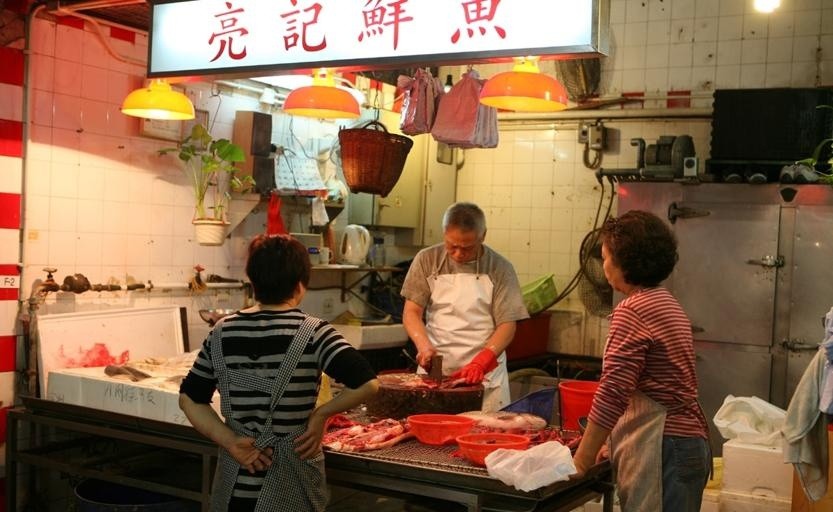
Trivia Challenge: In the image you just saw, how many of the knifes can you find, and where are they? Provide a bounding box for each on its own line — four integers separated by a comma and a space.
426, 355, 442, 387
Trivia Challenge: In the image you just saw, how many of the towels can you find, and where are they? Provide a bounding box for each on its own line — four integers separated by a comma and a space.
483, 441, 578, 493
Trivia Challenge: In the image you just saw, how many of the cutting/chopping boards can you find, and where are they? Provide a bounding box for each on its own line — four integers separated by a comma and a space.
365, 372, 484, 421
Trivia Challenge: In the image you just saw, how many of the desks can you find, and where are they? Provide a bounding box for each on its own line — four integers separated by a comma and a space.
6, 406, 619, 512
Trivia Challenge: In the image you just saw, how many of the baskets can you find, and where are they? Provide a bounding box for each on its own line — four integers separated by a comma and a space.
520, 273, 558, 315
339, 121, 414, 198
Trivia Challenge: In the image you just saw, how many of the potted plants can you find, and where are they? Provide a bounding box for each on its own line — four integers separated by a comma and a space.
158, 125, 257, 245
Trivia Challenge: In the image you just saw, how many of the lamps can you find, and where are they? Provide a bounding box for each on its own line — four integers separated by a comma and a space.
120, 74, 196, 122
283, 69, 362, 121
479, 56, 568, 113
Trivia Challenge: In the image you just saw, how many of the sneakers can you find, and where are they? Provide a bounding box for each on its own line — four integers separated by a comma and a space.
795, 163, 819, 184
723, 167, 743, 182
743, 166, 768, 183
780, 165, 795, 182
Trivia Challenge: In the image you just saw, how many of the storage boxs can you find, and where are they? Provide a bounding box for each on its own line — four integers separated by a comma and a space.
717, 490, 792, 512
720, 440, 798, 500
505, 312, 552, 361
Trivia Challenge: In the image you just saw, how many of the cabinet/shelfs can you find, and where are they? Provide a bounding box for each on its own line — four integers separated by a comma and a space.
347, 104, 425, 229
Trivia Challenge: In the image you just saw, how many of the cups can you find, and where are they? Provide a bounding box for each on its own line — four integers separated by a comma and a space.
319, 247, 333, 266
307, 247, 321, 267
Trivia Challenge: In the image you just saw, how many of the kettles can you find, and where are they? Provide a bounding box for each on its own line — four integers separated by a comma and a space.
339, 225, 370, 266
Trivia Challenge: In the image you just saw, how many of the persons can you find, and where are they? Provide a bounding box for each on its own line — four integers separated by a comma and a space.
178, 234, 379, 512
567, 211, 715, 512
399, 203, 531, 415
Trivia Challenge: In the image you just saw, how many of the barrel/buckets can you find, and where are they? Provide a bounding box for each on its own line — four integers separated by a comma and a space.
557, 378, 599, 432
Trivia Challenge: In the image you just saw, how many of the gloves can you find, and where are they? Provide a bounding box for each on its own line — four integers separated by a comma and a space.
452, 349, 499, 386
